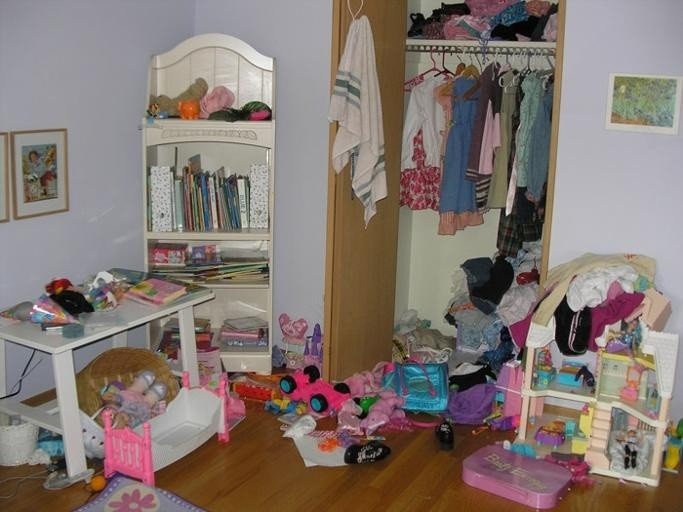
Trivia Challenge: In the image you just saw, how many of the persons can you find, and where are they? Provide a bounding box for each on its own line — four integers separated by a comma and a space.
22, 149, 54, 196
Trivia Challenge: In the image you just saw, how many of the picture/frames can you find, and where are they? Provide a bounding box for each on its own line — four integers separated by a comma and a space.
10, 127, 70, 220
604, 71, 682, 135
0, 131, 10, 222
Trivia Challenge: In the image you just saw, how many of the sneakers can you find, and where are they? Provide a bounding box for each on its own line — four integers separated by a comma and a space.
341, 439, 392, 464
433, 421, 456, 451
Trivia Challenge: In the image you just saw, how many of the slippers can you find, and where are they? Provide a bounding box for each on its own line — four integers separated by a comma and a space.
42, 465, 97, 491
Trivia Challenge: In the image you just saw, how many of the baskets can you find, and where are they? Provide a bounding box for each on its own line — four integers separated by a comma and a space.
73, 347, 180, 431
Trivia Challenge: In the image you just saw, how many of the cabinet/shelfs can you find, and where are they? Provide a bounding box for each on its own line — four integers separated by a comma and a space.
518, 332, 678, 487
322, 1, 567, 382
139, 33, 277, 377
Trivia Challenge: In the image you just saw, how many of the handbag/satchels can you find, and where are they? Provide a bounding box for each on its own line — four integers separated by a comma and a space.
382, 356, 451, 412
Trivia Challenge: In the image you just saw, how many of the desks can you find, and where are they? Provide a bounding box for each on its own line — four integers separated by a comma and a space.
0, 267, 216, 478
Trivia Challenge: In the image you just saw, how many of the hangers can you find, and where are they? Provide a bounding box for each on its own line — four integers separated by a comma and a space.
402, 45, 557, 96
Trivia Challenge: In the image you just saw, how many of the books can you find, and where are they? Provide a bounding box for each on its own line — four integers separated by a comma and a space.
123, 242, 266, 306
144, 150, 266, 231
153, 315, 266, 352
